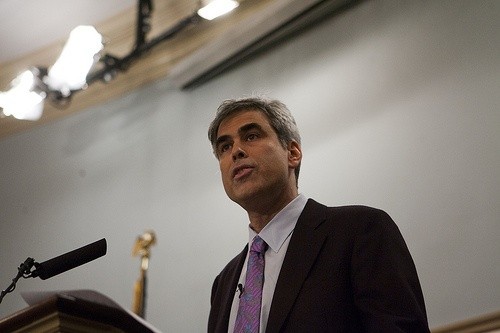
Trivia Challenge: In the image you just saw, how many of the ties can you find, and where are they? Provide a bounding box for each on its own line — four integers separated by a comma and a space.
232, 235, 268, 333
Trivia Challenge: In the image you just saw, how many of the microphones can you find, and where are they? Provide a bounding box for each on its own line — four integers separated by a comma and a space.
31, 238, 107, 280
238, 284, 244, 298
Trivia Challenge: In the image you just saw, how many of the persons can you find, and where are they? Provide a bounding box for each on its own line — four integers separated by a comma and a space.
206, 95, 431, 333
